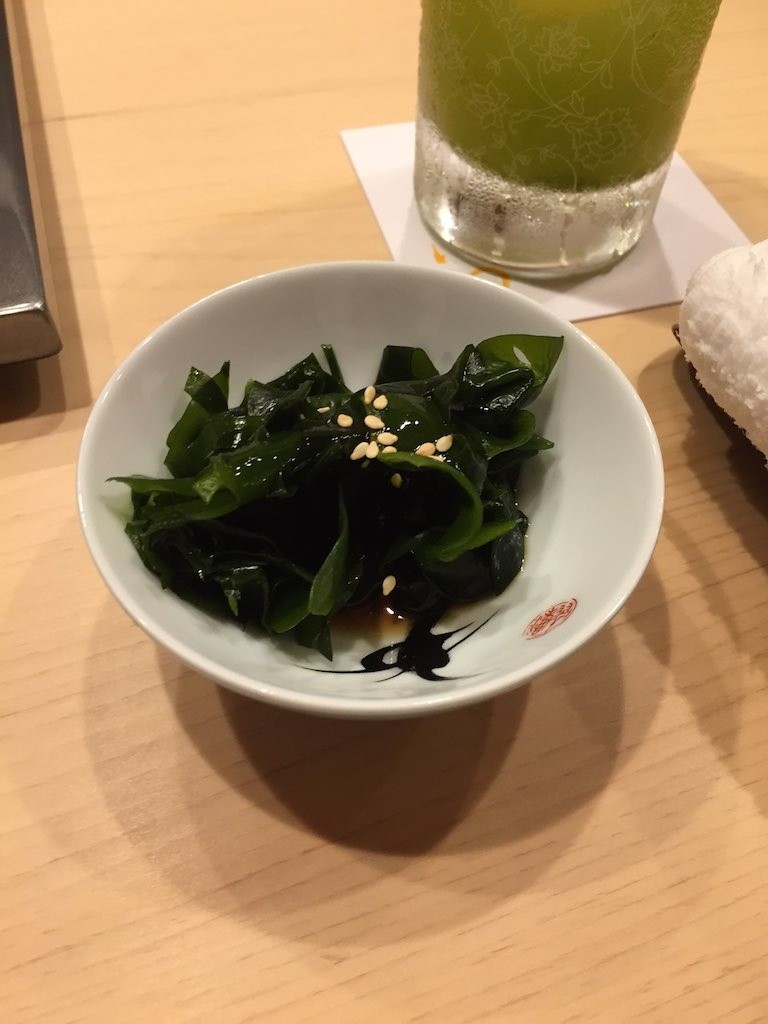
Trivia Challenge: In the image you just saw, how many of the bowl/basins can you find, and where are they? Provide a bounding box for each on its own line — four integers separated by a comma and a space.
78, 260, 666, 723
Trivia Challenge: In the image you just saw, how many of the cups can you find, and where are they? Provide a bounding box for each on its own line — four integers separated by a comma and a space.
413, 3, 718, 282
